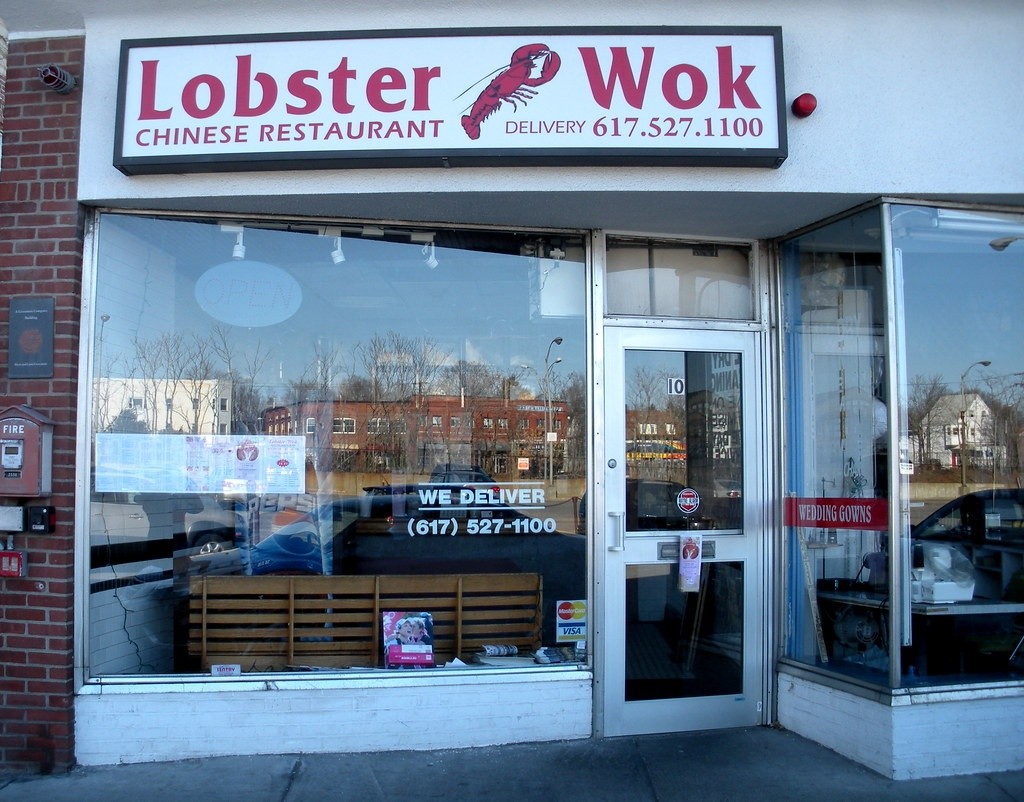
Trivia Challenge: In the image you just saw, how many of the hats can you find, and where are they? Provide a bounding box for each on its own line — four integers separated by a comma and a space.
396, 618, 412, 629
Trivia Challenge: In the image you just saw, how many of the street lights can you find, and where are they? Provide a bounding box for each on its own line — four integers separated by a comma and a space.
520, 358, 563, 480
545, 336, 564, 486
960, 360, 1001, 498
987, 236, 1024, 253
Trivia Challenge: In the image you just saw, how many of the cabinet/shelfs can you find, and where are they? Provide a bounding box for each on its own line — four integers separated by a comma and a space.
959, 541, 1024, 601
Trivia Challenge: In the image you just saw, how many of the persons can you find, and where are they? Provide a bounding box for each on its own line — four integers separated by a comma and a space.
384, 612, 434, 669
133, 491, 204, 540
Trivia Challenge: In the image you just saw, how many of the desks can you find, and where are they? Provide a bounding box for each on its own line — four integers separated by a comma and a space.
807, 542, 844, 580
816, 589, 1024, 676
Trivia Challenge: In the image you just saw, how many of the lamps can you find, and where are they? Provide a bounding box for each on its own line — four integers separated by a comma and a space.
218, 219, 439, 269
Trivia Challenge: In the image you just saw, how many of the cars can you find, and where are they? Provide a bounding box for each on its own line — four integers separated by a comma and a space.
578, 478, 702, 532
90, 463, 587, 651
713, 479, 741, 499
878, 488, 1024, 602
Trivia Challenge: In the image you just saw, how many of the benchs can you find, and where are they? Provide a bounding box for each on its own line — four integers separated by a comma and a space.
189, 571, 544, 675
